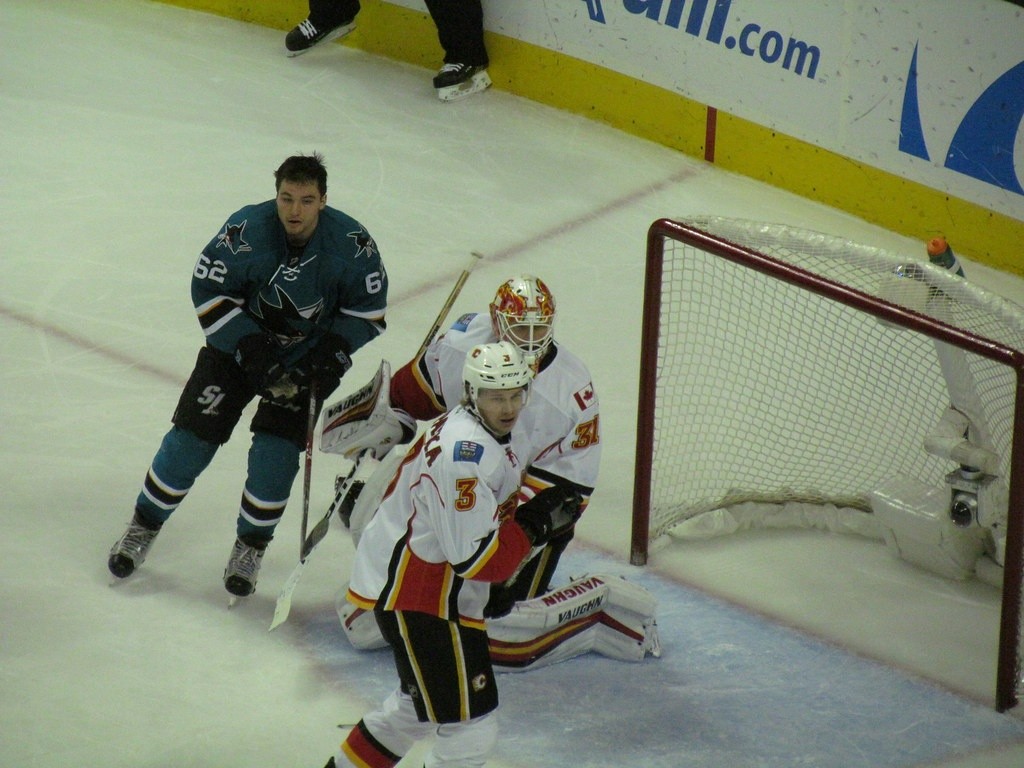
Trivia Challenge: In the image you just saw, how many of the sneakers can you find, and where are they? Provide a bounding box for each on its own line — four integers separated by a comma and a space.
433, 62, 493, 101
108, 509, 164, 585
285, 16, 357, 58
223, 540, 266, 607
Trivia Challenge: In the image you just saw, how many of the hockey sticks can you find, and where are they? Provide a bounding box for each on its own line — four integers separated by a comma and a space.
336, 482, 581, 732
266, 246, 484, 633
297, 376, 331, 562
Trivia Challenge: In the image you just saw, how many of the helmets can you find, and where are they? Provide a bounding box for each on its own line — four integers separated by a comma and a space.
490, 277, 555, 362
462, 341, 534, 391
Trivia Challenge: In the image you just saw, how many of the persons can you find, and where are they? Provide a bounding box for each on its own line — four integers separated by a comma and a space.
322, 341, 583, 767
319, 275, 659, 674
286, 0, 489, 89
107, 151, 388, 598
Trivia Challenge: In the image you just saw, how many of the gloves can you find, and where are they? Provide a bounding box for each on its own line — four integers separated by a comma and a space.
290, 333, 351, 387
254, 363, 305, 411
483, 582, 522, 620
514, 483, 584, 547
